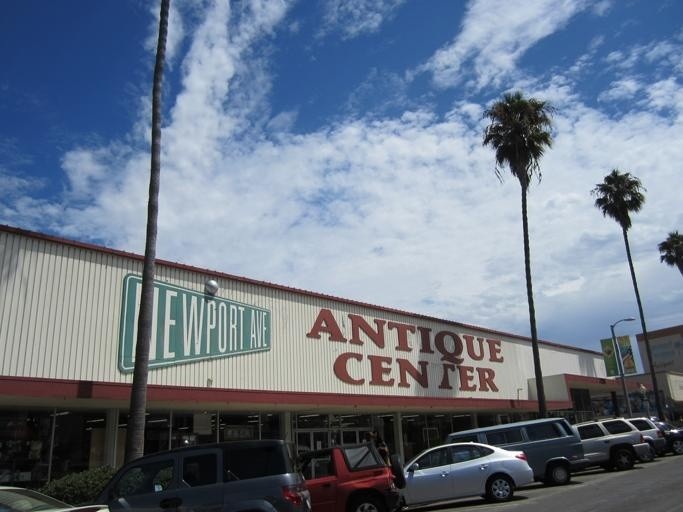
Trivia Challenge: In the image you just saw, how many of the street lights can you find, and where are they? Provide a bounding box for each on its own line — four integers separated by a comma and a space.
608, 316, 637, 418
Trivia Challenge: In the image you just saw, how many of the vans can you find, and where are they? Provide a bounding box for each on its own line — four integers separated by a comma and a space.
446, 418, 585, 484
88, 438, 310, 510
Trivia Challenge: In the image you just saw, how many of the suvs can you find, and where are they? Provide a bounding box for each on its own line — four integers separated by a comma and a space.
298, 441, 407, 512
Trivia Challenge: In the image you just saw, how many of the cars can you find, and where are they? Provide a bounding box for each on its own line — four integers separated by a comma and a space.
573, 414, 682, 470
398, 442, 535, 507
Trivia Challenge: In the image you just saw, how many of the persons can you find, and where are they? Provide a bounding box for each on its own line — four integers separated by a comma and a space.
372, 430, 390, 467
360, 431, 373, 444
